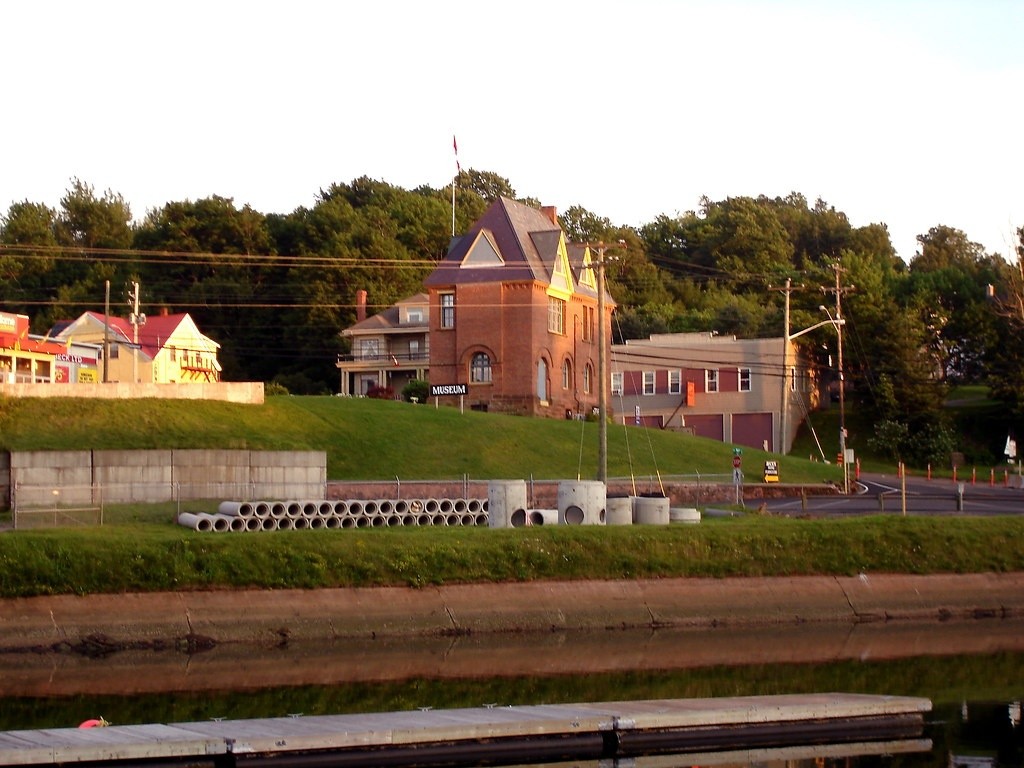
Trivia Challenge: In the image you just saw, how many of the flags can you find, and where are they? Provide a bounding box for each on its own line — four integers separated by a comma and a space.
392, 354, 399, 367
457, 161, 460, 170
16, 327, 75, 351
453, 136, 458, 155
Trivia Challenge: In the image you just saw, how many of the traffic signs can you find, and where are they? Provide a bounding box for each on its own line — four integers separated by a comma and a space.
763, 460, 780, 484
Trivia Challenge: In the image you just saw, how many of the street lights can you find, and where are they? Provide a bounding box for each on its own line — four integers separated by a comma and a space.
779, 317, 847, 458
819, 305, 853, 494
